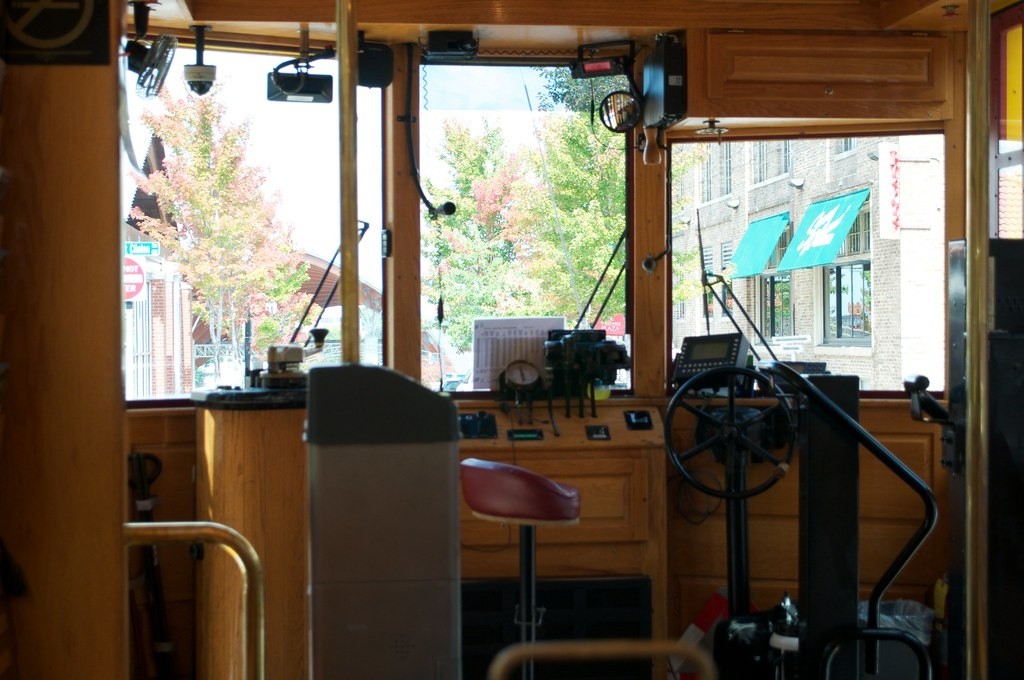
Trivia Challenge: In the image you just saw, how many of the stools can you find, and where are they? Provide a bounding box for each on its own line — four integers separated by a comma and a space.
460, 456, 580, 680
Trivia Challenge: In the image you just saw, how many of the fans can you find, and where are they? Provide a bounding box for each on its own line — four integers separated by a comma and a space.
124, 0, 177, 96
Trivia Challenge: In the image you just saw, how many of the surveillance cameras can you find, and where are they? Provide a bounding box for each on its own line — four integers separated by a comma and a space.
183, 65, 217, 96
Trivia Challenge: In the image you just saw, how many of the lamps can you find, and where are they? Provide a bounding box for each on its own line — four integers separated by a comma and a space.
787, 179, 805, 189
725, 199, 740, 208
679, 216, 691, 224
868, 151, 879, 161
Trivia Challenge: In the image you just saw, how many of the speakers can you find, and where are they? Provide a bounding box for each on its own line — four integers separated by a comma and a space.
355, 42, 395, 88
